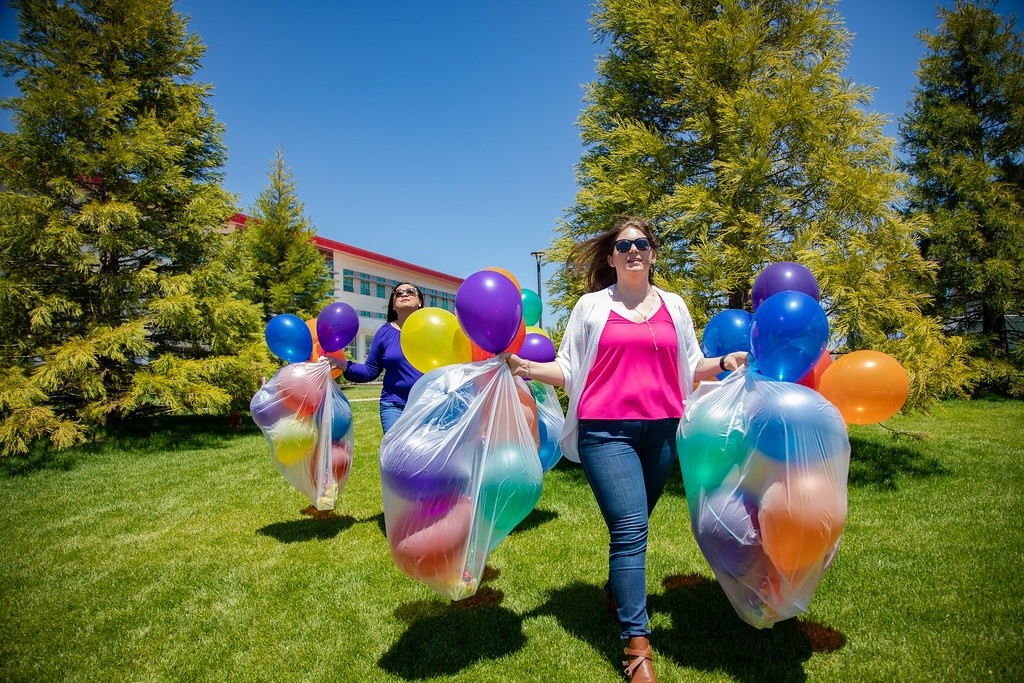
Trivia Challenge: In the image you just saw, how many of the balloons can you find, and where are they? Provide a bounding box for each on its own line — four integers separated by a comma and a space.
312, 343, 345, 377
316, 302, 359, 352
701, 309, 752, 380
796, 351, 832, 393
473, 321, 526, 361
519, 289, 542, 326
752, 262, 818, 309
487, 268, 521, 296
250, 363, 352, 508
751, 291, 829, 384
401, 307, 472, 375
306, 319, 320, 343
819, 350, 909, 424
516, 326, 556, 381
454, 270, 521, 357
677, 362, 849, 625
266, 314, 313, 363
381, 368, 564, 574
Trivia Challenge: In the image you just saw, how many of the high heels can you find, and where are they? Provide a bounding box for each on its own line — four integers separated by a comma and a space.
622, 644, 656, 683
604, 580, 624, 625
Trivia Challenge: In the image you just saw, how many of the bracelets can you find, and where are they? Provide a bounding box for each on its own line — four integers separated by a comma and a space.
720, 354, 731, 371
523, 359, 530, 378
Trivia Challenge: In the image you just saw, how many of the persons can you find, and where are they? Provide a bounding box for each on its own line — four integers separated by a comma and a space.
505, 216, 747, 683
318, 283, 424, 438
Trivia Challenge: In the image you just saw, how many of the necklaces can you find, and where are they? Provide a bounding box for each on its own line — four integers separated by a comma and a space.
616, 286, 658, 351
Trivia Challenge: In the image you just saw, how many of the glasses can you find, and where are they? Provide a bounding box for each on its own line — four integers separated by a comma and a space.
392, 288, 419, 297
614, 237, 652, 253
